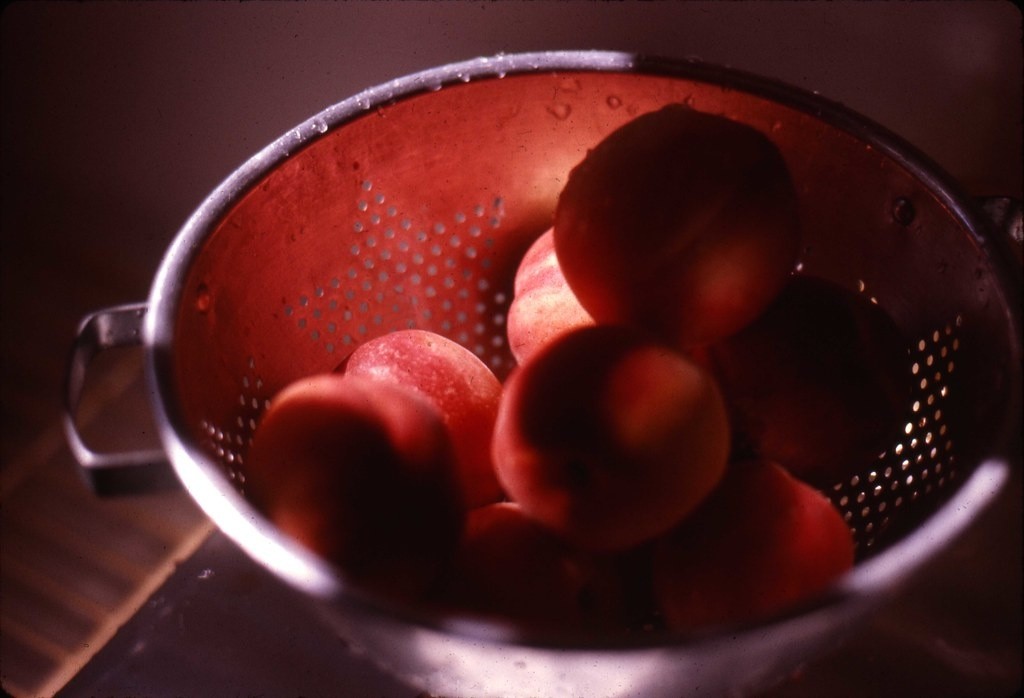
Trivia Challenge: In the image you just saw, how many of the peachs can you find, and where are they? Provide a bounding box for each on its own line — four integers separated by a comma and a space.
246, 104, 904, 650
